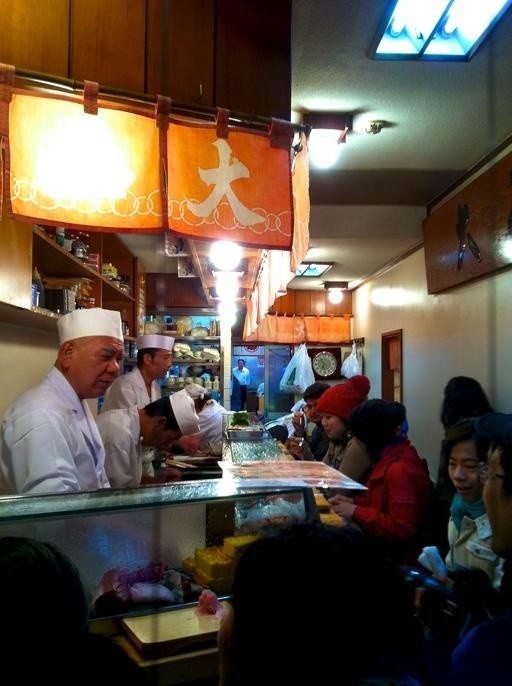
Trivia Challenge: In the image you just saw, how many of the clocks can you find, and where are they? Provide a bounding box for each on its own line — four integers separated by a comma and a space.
307, 348, 342, 381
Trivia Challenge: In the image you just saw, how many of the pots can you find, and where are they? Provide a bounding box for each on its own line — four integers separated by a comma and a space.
264, 420, 288, 445
122, 320, 128, 334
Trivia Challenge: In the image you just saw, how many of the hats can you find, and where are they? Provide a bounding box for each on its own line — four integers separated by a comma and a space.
314, 374, 371, 422
343, 397, 407, 449
55, 306, 124, 347
168, 387, 201, 437
133, 334, 176, 353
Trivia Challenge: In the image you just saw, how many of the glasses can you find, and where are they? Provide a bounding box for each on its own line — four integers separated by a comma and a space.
477, 464, 505, 486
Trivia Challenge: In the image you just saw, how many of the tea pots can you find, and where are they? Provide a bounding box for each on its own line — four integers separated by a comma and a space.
69, 232, 89, 258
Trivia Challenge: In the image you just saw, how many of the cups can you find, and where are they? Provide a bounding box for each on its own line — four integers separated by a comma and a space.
55, 225, 66, 247
259, 392, 265, 412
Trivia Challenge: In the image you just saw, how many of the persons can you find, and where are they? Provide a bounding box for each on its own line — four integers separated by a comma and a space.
1, 306, 510, 685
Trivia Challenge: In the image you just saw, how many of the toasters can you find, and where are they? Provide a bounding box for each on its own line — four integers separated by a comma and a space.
124, 341, 129, 360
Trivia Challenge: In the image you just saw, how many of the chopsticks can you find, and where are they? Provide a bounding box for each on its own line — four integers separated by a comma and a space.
299, 437, 305, 447
300, 408, 303, 425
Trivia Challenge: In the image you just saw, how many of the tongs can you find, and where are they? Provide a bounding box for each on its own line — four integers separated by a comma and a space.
164, 459, 197, 469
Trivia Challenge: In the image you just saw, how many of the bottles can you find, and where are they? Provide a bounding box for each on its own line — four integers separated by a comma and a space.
102, 256, 132, 297
164, 374, 220, 392
85, 298, 96, 309
31, 266, 45, 310
131, 342, 138, 358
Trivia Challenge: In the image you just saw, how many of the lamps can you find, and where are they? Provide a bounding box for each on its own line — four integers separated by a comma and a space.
368, 0, 511, 63
295, 261, 335, 278
294, 107, 369, 169
322, 281, 348, 305
